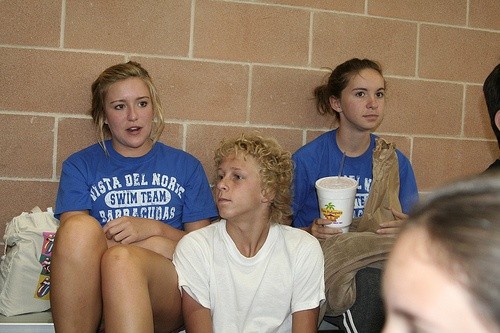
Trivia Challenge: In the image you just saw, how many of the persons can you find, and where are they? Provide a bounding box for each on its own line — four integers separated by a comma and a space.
379, 172, 499, 333
49, 60, 220, 333
290, 58, 422, 333
171, 128, 325, 333
478, 63, 500, 174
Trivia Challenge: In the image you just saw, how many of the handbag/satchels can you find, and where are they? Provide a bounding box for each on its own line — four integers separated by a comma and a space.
0, 207, 59, 317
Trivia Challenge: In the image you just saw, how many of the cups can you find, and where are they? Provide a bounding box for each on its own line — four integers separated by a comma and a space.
315, 176, 358, 233
